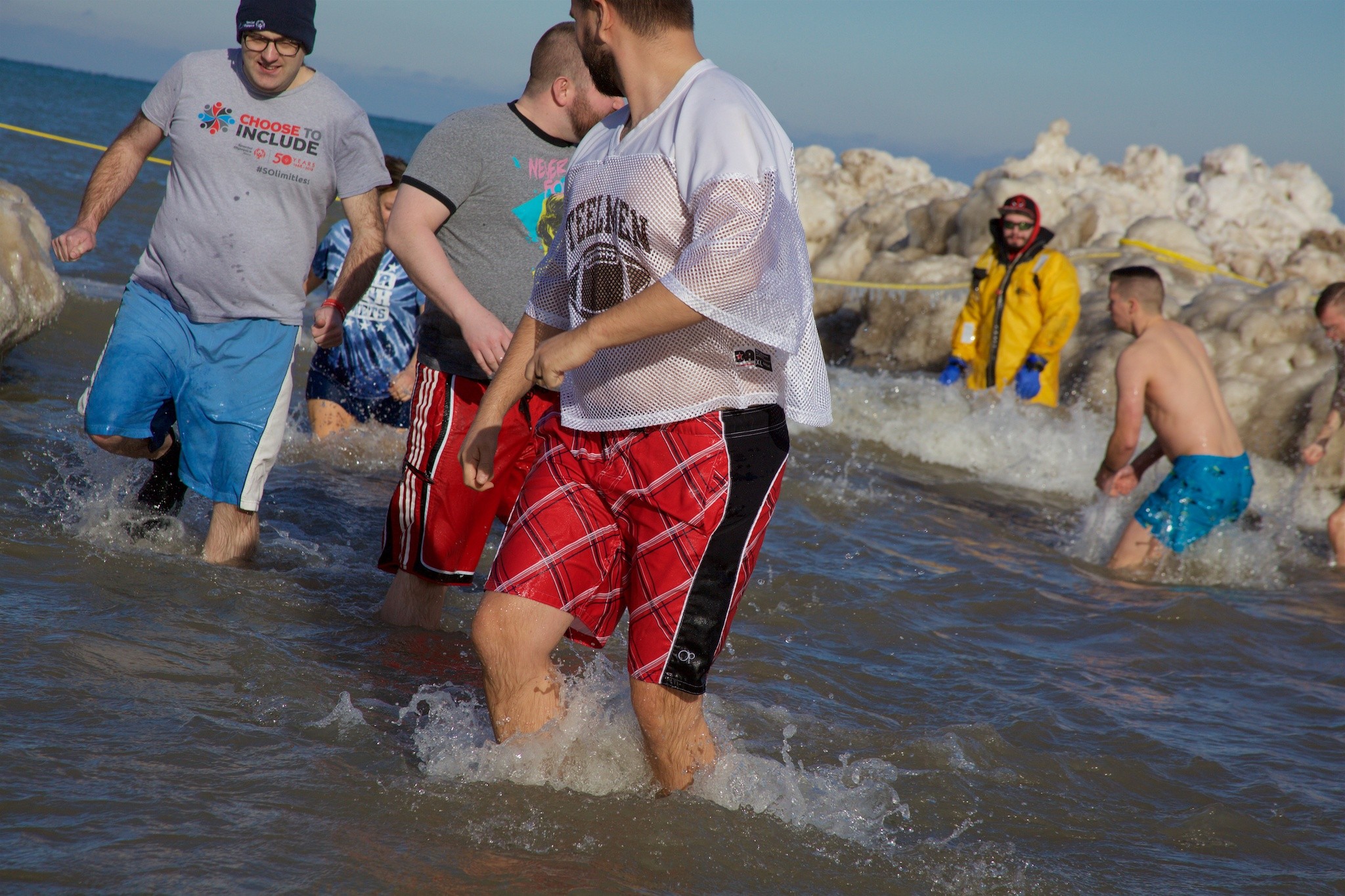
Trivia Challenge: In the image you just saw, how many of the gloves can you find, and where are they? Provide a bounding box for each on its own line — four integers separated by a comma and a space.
939, 354, 967, 385
1014, 356, 1047, 399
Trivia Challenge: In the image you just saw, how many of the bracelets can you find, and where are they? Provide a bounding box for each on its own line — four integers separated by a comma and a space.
321, 299, 347, 323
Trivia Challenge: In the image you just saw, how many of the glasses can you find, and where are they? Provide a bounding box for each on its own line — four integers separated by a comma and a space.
241, 30, 302, 57
1001, 219, 1034, 230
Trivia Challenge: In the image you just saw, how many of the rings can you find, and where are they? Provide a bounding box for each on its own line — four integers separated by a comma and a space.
535, 376, 542, 379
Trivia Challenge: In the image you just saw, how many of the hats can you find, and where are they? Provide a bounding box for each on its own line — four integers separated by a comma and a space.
998, 196, 1036, 219
236, 0, 317, 55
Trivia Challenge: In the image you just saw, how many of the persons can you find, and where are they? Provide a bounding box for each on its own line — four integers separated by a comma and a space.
378, 20, 625, 634
1297, 282, 1344, 568
304, 155, 427, 438
941, 194, 1079, 407
51, 0, 395, 567
1096, 266, 1255, 566
455, 1, 835, 797
